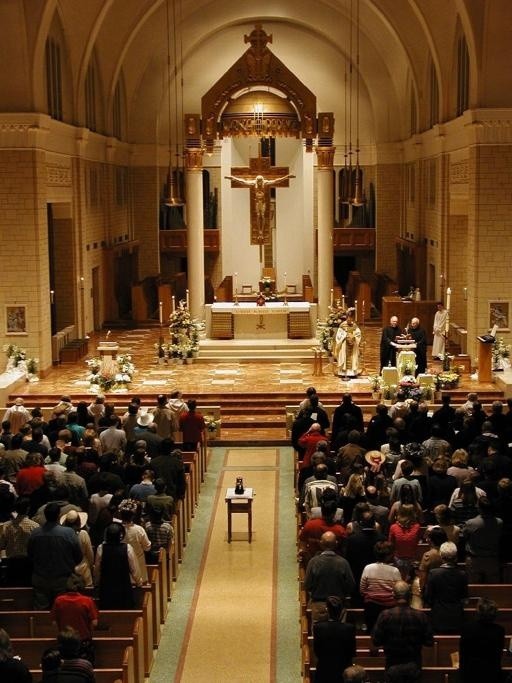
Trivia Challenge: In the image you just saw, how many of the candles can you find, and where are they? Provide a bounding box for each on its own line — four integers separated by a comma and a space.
355, 301, 357, 321
172, 296, 175, 312
342, 295, 344, 310
186, 290, 190, 308
331, 289, 333, 308
446, 287, 451, 310
159, 302, 163, 323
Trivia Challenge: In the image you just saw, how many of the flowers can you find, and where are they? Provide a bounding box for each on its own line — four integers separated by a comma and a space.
155, 307, 206, 358
493, 337, 512, 360
382, 386, 393, 401
422, 352, 463, 391
369, 374, 384, 392
3, 343, 38, 384
320, 307, 354, 351
395, 374, 423, 401
84, 355, 136, 395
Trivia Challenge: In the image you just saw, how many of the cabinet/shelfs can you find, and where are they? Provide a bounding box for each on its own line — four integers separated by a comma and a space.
227, 487, 253, 543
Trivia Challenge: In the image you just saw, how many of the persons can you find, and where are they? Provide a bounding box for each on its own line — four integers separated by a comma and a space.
388, 390, 411, 419
336, 429, 367, 487
431, 302, 450, 361
303, 463, 340, 520
297, 422, 330, 469
178, 398, 205, 473
381, 426, 405, 456
344, 463, 366, 504
39, 647, 65, 683
316, 439, 337, 476
458, 598, 505, 683
421, 541, 468, 635
332, 393, 365, 443
380, 435, 404, 480
92, 523, 144, 610
26, 502, 84, 611
118, 498, 152, 586
298, 500, 347, 555
145, 504, 176, 565
299, 386, 324, 412
347, 502, 382, 547
388, 483, 425, 524
89, 507, 113, 554
418, 527, 449, 590
50, 573, 99, 669
461, 495, 504, 584
0, 495, 40, 559
297, 450, 326, 494
57, 631, 97, 683
298, 393, 331, 438
310, 488, 345, 523
391, 442, 435, 501
0, 387, 188, 522
405, 317, 427, 377
389, 460, 423, 509
60, 510, 94, 596
388, 503, 421, 583
424, 503, 460, 543
0, 626, 35, 683
423, 391, 512, 509
365, 404, 394, 451
303, 530, 358, 608
312, 595, 368, 683
359, 540, 403, 636
379, 315, 402, 376
365, 485, 389, 536
224, 173, 297, 240
370, 580, 435, 683
335, 310, 363, 377
403, 400, 428, 443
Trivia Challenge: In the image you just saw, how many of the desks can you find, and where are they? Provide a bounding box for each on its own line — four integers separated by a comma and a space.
210, 303, 312, 338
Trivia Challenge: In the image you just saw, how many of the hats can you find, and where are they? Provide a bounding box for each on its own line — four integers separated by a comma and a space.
137, 410, 155, 428
364, 449, 386, 466
406, 442, 425, 459
58, 509, 88, 529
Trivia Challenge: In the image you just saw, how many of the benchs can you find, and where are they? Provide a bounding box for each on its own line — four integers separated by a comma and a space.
175, 420, 211, 578
296, 566, 481, 596
146, 547, 169, 626
0, 645, 136, 683
301, 616, 462, 666
1, 569, 161, 650
0, 616, 146, 683
1, 592, 155, 678
302, 645, 462, 683
297, 590, 481, 628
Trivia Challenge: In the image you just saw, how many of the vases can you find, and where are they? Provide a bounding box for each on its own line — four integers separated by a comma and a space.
174, 357, 180, 363
167, 358, 175, 365
383, 399, 392, 405
372, 393, 380, 400
158, 358, 163, 364
495, 359, 506, 371
176, 359, 183, 365
186, 358, 194, 364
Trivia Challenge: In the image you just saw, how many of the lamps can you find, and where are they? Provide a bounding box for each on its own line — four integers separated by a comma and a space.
177, 1, 185, 208
165, 0, 177, 209
351, 0, 365, 207
338, 0, 351, 205
